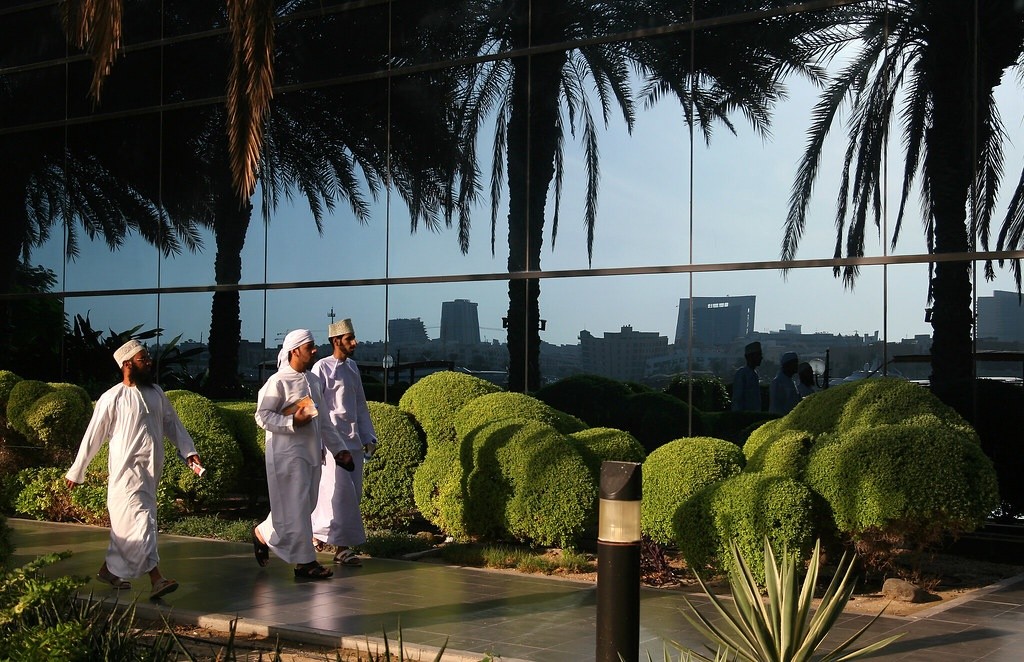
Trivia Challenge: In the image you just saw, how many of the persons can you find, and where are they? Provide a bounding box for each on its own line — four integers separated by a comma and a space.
64, 339, 204, 600
309, 317, 378, 567
250, 328, 356, 580
726, 340, 816, 416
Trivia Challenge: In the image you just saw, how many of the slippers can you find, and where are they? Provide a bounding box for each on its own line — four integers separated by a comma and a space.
294, 560, 334, 579
96, 573, 131, 588
251, 526, 269, 567
312, 533, 323, 552
332, 548, 362, 566
149, 578, 179, 599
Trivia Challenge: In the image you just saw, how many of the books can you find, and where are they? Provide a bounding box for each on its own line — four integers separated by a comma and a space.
176, 449, 207, 477
281, 395, 319, 427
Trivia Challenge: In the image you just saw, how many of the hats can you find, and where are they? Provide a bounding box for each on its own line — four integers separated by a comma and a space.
328, 318, 355, 338
113, 340, 147, 368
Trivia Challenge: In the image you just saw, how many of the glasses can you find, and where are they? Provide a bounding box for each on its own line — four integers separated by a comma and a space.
129, 355, 152, 364
